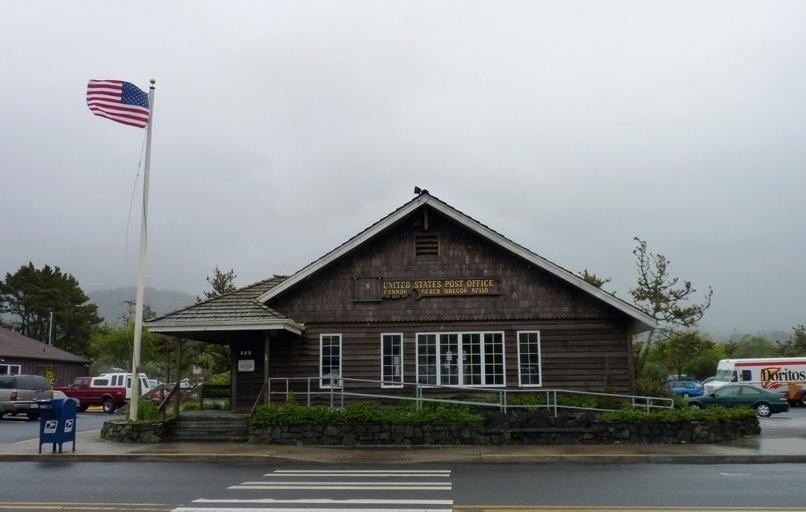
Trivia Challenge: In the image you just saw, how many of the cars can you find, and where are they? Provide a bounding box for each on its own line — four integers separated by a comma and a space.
661, 358, 805, 418
0, 364, 204, 420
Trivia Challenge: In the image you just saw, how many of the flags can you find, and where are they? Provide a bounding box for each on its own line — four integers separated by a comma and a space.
86, 79, 149, 128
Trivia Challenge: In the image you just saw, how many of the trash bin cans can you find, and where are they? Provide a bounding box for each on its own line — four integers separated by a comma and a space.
35, 397, 76, 454
